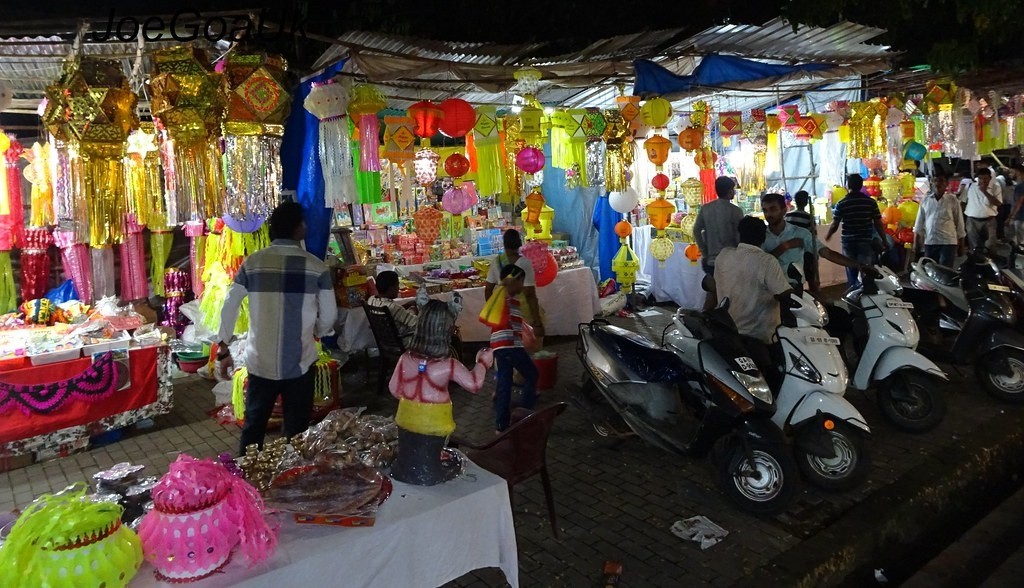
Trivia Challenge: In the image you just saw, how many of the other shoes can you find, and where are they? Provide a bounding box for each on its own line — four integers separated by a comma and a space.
496, 428, 504, 435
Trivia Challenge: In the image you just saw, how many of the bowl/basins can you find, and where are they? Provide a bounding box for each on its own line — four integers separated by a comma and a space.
176, 352, 209, 373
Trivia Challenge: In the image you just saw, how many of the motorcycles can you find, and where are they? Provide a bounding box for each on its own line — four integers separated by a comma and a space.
910, 202, 1024, 333
739, 260, 876, 495
784, 236, 951, 434
841, 225, 1023, 407
564, 296, 801, 515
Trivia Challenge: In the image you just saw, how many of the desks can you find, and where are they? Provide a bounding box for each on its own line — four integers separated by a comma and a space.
126, 447, 519, 588
377, 248, 602, 340
0, 344, 174, 472
643, 222, 847, 311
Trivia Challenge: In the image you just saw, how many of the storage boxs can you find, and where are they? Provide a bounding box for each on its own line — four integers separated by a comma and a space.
92, 428, 121, 446
0, 324, 132, 370
335, 264, 368, 286
552, 233, 569, 240
333, 285, 368, 307
0, 453, 32, 472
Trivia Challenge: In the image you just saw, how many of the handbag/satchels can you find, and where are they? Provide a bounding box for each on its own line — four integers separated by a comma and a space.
478, 274, 514, 330
521, 321, 543, 353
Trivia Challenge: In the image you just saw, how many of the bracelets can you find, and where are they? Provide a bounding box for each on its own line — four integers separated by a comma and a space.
218, 349, 229, 360
533, 322, 542, 327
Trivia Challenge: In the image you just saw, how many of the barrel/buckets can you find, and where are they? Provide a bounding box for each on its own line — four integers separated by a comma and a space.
532, 357, 558, 389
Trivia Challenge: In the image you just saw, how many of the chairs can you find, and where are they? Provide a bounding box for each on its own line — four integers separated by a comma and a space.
450, 402, 567, 560
360, 299, 405, 380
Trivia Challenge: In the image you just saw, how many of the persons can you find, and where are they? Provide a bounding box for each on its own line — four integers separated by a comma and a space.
490, 264, 539, 433
389, 299, 494, 485
693, 163, 1024, 356
485, 229, 545, 337
217, 201, 337, 459
368, 271, 417, 348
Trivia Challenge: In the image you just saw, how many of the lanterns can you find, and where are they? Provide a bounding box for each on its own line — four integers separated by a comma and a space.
40, 44, 993, 290
